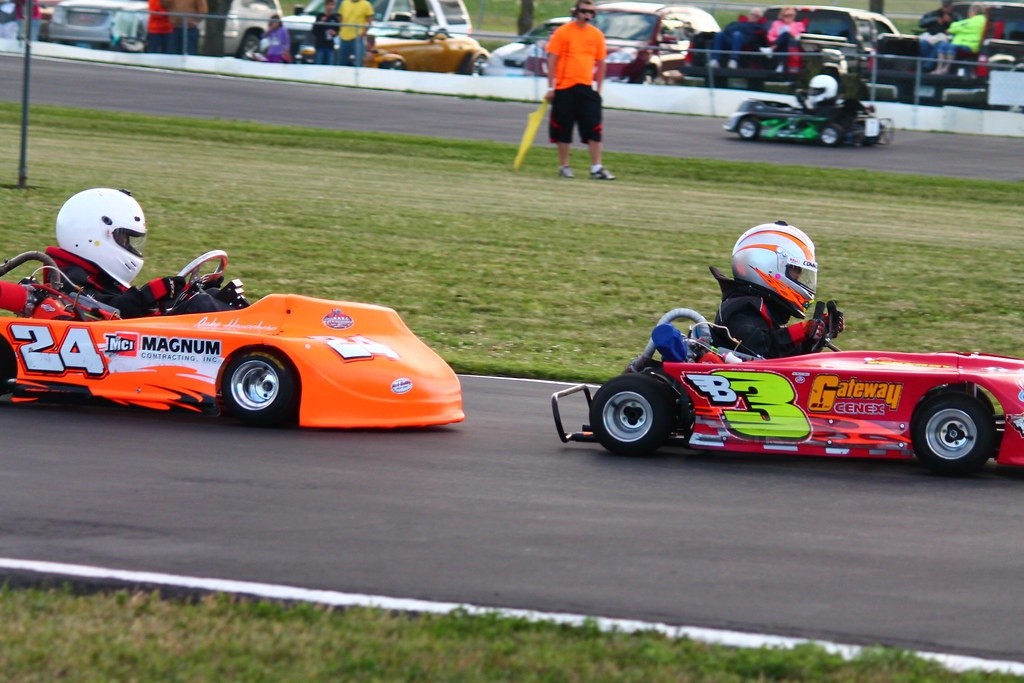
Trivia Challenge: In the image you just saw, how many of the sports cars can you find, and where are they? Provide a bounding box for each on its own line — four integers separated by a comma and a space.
333, 25, 490, 77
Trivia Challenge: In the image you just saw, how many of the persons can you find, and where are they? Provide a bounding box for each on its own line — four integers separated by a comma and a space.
311, 0, 342, 65
41, 188, 236, 319
13, 0, 42, 41
914, 0, 988, 75
146, 0, 209, 55
545, 0, 616, 180
759, 6, 805, 73
710, 221, 844, 359
803, 74, 838, 111
337, 0, 375, 66
260, 15, 291, 63
706, 8, 763, 69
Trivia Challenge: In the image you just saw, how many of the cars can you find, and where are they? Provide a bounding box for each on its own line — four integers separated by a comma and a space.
524, 2, 721, 84
0, 0, 283, 60
478, 17, 574, 77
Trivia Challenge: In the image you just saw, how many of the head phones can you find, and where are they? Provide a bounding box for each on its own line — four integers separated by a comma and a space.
569, 7, 597, 18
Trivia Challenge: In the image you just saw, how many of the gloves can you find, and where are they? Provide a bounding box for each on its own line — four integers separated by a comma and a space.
802, 312, 844, 340
142, 275, 186, 306
175, 273, 223, 309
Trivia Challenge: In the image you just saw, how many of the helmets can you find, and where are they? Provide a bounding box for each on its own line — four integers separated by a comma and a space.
57, 189, 147, 290
808, 75, 839, 105
732, 222, 817, 305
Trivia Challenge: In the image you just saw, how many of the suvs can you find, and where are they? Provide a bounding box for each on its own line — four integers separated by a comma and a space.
277, 1, 473, 63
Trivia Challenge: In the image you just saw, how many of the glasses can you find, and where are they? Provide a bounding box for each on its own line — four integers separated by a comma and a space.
578, 7, 595, 16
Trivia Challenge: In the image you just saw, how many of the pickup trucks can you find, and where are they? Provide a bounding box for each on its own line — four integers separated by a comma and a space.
679, 4, 902, 84
867, 0, 1024, 111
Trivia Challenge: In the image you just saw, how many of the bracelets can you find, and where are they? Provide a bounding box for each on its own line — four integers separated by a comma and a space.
547, 88, 555, 91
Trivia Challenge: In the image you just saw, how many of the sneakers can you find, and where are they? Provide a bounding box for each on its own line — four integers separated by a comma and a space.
588, 169, 613, 180
557, 166, 573, 178
